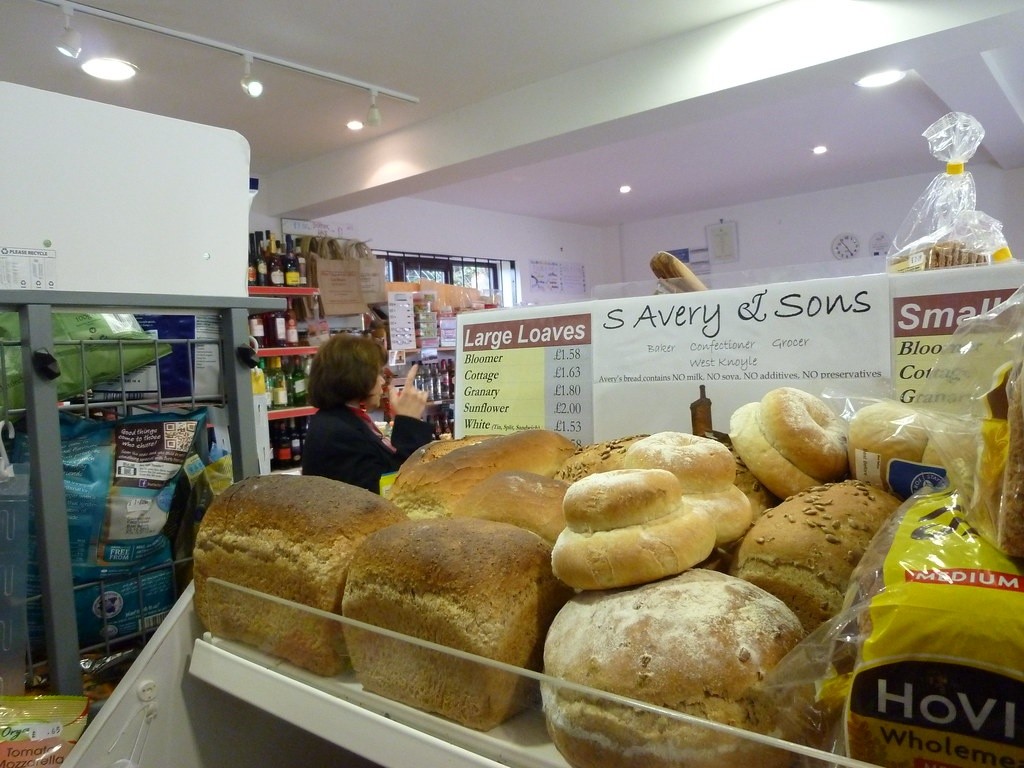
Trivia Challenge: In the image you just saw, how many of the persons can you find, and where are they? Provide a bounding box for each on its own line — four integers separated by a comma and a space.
302, 334, 433, 496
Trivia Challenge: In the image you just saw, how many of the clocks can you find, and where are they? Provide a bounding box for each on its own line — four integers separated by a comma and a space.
830, 233, 861, 260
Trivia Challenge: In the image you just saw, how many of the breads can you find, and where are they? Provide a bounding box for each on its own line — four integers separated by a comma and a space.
890, 237, 991, 272
190, 367, 1024, 768
649, 251, 709, 293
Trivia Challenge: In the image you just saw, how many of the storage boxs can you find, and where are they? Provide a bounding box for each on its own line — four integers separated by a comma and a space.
415, 327, 438, 337
439, 339, 456, 347
438, 328, 456, 339
436, 317, 456, 330
416, 337, 440, 348
415, 320, 438, 328
414, 311, 438, 320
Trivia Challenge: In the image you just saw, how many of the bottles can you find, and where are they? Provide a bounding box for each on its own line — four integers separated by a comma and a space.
271, 415, 313, 471
249, 297, 298, 348
426, 408, 454, 440
411, 358, 455, 401
248, 229, 308, 287
259, 355, 312, 410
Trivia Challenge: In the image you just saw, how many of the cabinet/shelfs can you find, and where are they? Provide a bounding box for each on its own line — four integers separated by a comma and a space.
248, 284, 322, 474
362, 303, 454, 406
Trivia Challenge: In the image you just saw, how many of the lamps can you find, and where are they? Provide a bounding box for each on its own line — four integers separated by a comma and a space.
54, 24, 82, 58
366, 104, 381, 127
240, 76, 264, 99
